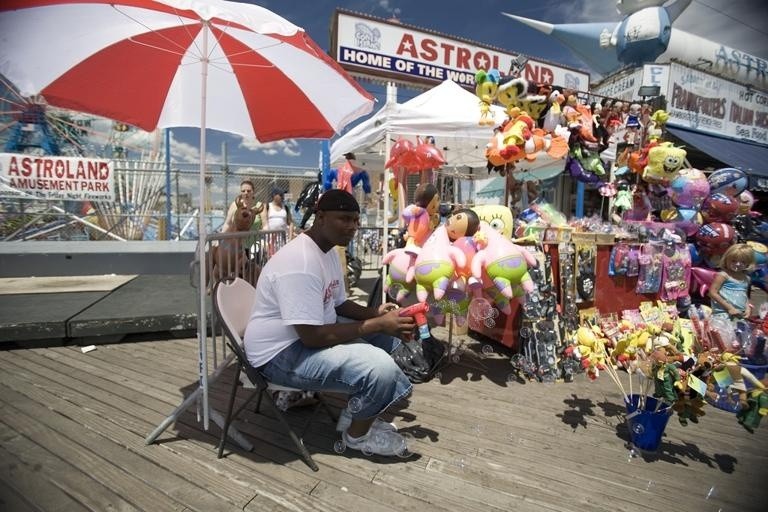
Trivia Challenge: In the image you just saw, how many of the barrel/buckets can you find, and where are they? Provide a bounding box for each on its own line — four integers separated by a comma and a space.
623, 394, 674, 453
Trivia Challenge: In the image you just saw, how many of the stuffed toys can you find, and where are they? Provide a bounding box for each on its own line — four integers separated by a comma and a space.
475, 71, 687, 190
566, 321, 750, 427
383, 182, 535, 339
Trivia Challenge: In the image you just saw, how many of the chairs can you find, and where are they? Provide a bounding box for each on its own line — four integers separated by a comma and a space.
211, 275, 351, 471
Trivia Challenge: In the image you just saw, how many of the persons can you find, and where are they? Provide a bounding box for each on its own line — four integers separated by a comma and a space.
707, 244, 755, 328
243, 190, 417, 461
200, 181, 269, 295
265, 189, 294, 252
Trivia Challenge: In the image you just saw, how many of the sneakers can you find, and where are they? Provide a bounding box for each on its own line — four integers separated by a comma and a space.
336, 408, 405, 456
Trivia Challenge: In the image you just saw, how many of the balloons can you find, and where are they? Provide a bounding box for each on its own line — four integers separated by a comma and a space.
662, 168, 754, 256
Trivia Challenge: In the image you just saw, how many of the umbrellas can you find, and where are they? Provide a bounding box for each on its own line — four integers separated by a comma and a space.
1, 0, 376, 432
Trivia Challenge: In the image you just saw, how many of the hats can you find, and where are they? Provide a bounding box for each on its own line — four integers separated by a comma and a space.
270, 188, 287, 196
317, 189, 359, 212
